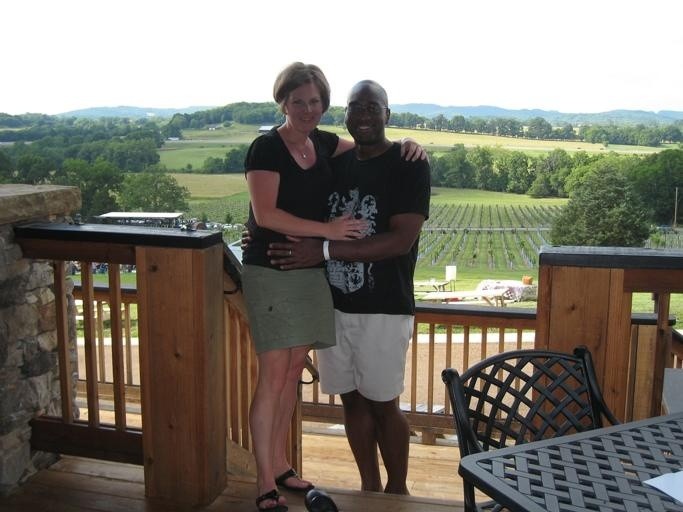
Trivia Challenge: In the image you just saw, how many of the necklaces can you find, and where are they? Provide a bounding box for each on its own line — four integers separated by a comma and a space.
286, 132, 310, 159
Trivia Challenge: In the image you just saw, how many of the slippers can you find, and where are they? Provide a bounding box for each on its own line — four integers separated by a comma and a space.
256, 466, 314, 512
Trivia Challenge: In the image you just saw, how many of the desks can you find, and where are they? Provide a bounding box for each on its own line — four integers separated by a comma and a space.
459, 410, 682, 508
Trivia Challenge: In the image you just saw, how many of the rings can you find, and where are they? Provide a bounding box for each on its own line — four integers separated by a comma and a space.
288, 249, 292, 257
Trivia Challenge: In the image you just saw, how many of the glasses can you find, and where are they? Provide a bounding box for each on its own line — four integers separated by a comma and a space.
348, 105, 386, 115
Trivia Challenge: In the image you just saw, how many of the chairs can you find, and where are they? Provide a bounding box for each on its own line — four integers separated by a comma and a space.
442, 347, 621, 453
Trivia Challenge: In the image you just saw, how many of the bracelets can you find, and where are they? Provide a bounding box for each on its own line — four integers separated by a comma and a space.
323, 240, 331, 260
